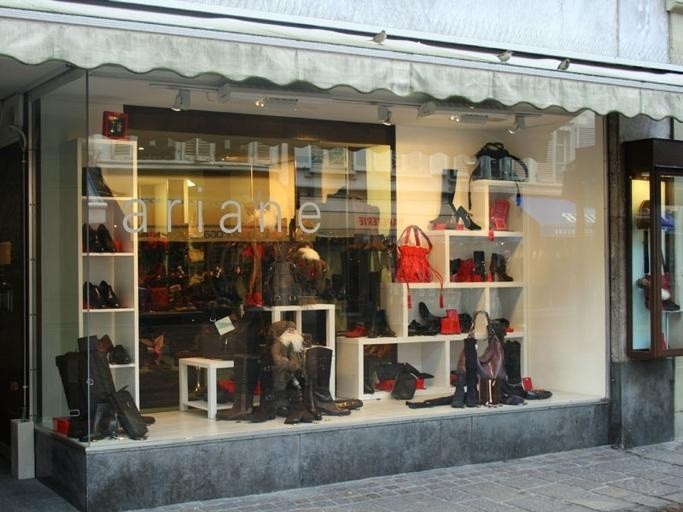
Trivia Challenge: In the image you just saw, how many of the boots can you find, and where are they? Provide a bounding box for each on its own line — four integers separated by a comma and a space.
382, 318, 395, 337
428, 168, 457, 225
283, 346, 365, 425
480, 341, 553, 406
464, 339, 478, 407
490, 251, 514, 282
474, 251, 485, 279
451, 371, 464, 408
55, 351, 149, 442
368, 315, 380, 338
454, 258, 473, 282
216, 354, 252, 419
252, 363, 278, 424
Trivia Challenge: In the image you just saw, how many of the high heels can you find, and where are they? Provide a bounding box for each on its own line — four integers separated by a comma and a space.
99, 282, 121, 308
455, 206, 483, 230
82, 222, 99, 252
97, 334, 113, 364
84, 282, 99, 309
97, 225, 116, 252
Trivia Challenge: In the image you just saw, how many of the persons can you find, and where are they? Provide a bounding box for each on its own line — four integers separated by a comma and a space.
268, 317, 312, 392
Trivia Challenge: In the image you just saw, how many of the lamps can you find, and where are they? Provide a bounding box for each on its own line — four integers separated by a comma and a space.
168, 87, 531, 135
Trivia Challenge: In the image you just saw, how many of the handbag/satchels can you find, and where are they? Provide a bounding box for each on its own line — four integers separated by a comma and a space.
488, 198, 510, 242
395, 226, 444, 310
457, 311, 508, 379
471, 141, 529, 181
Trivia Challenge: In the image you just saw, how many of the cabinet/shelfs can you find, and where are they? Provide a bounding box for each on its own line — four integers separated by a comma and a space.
64, 136, 141, 421
260, 303, 337, 412
334, 178, 532, 404
180, 359, 235, 424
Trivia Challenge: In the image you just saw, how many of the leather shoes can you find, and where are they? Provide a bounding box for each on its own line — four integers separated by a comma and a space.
140, 242, 270, 313
343, 322, 370, 340
362, 354, 436, 399
109, 342, 131, 364
